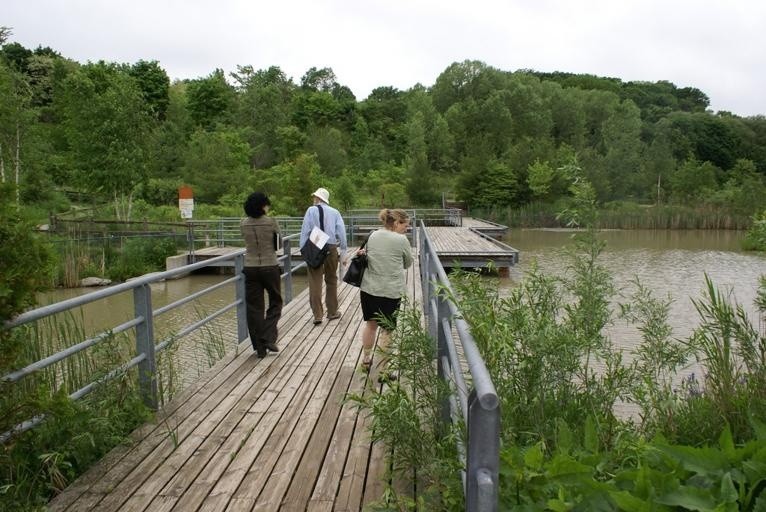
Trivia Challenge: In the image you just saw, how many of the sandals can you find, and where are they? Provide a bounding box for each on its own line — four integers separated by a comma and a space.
362, 361, 371, 371
378, 370, 397, 383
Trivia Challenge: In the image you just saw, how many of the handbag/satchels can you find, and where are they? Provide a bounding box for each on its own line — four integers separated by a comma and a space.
298, 238, 329, 269
343, 250, 366, 287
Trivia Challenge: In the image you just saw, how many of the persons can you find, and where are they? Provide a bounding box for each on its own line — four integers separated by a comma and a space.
300, 188, 346, 323
360, 209, 413, 382
241, 192, 282, 357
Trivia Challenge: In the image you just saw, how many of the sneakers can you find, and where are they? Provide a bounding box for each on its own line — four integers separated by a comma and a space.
255, 344, 279, 357
328, 310, 341, 320
313, 317, 323, 324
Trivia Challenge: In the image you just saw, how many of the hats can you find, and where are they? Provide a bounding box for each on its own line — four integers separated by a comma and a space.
311, 188, 329, 204
